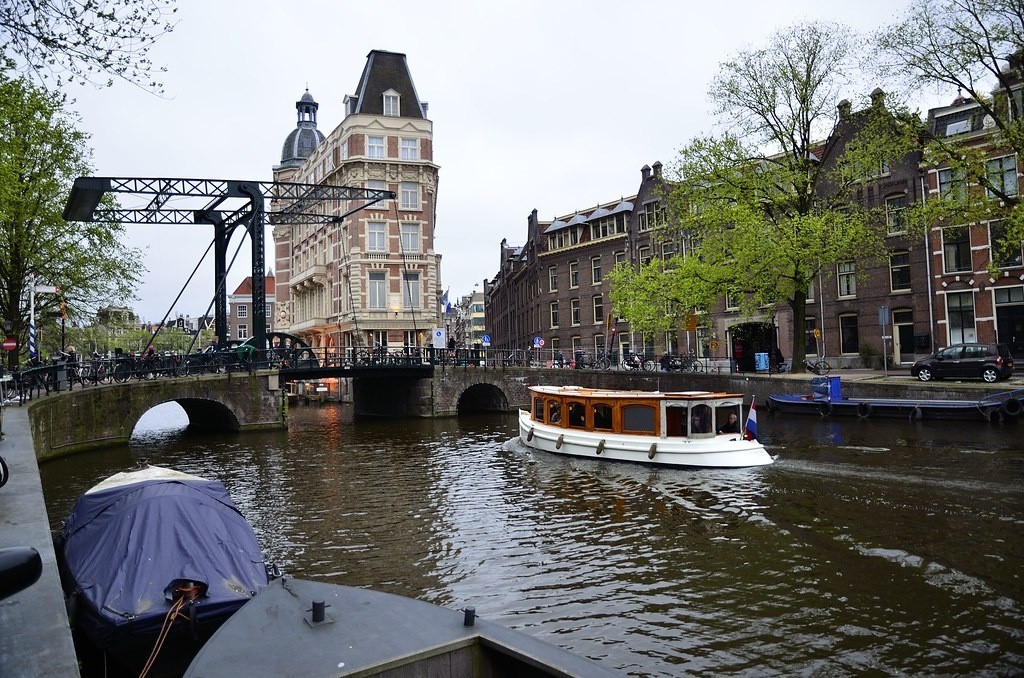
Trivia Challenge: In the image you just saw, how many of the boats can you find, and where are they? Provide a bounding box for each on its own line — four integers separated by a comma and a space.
58, 454, 283, 649
181, 561, 664, 678
768, 375, 1024, 426
515, 385, 774, 472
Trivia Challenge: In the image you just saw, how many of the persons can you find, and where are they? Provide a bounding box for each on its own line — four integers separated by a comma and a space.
733, 338, 743, 372
144, 346, 161, 371
690, 416, 705, 434
177, 345, 184, 356
524, 344, 533, 368
769, 345, 784, 374
549, 403, 603, 428
719, 414, 739, 434
65, 345, 78, 382
657, 352, 672, 372
557, 350, 563, 369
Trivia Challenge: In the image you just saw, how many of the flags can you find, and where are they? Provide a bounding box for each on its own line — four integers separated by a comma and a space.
746, 398, 758, 437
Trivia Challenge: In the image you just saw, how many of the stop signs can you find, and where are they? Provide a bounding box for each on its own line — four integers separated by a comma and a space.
610, 324, 616, 335
2, 337, 17, 351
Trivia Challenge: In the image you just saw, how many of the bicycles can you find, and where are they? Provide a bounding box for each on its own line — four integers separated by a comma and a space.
508, 347, 541, 368
582, 351, 704, 372
804, 358, 832, 375
769, 360, 791, 374
4, 348, 230, 398
355, 341, 393, 366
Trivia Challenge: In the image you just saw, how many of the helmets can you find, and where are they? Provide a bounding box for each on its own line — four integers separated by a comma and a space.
149, 345, 154, 350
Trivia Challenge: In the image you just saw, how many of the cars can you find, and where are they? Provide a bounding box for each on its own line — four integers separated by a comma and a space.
912, 343, 1015, 382
